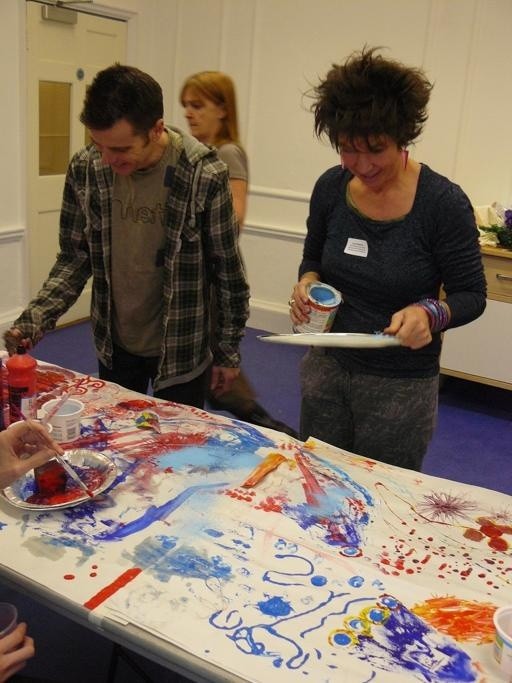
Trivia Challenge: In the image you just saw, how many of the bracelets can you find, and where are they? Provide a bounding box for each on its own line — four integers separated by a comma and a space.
418, 296, 449, 336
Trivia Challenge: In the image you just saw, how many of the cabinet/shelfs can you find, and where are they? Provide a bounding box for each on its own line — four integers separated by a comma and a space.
439, 250, 512, 386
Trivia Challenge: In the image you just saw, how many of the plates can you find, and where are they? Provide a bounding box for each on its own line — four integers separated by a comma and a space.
3, 447, 118, 509
257, 331, 403, 349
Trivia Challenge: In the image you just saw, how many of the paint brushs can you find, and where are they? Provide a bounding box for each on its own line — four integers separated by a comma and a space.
32, 378, 83, 423
10, 402, 94, 499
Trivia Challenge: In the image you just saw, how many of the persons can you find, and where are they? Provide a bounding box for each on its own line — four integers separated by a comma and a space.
285, 45, 487, 471
179, 69, 249, 234
4, 63, 251, 413
0, 419, 64, 680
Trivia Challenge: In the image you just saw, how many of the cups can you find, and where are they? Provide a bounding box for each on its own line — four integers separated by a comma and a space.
42, 399, 82, 443
495, 605, 512, 672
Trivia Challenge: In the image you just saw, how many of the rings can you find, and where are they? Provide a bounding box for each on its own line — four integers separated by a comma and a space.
288, 299, 293, 304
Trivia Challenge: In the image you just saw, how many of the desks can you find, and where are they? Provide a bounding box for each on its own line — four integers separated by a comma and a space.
0, 345, 511, 680
